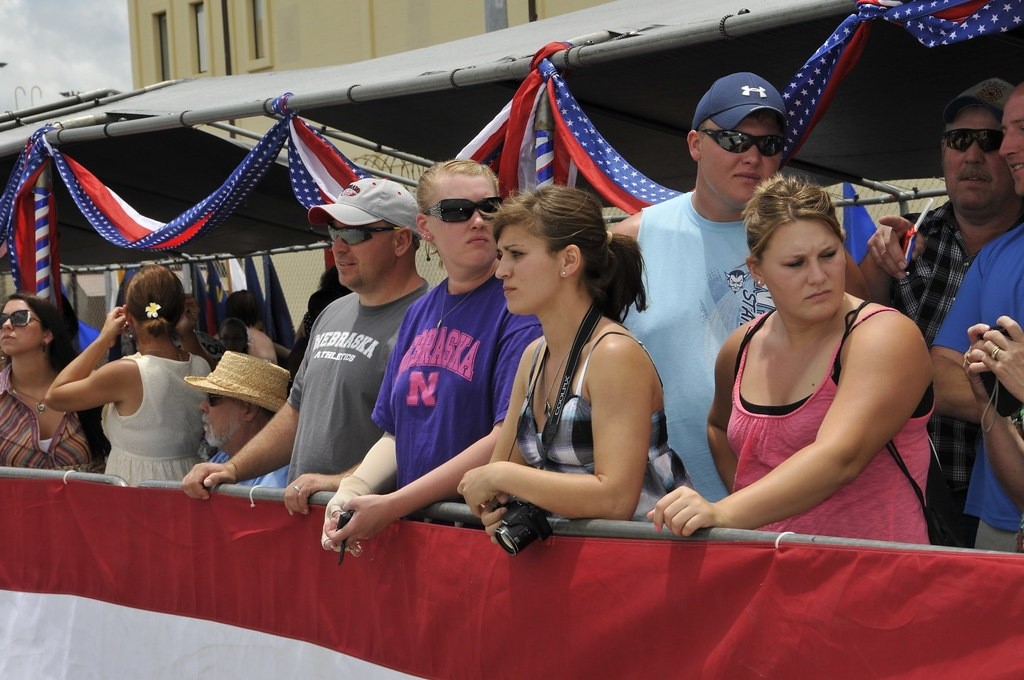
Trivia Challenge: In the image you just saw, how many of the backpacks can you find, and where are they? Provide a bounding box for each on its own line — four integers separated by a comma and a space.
737, 300, 972, 548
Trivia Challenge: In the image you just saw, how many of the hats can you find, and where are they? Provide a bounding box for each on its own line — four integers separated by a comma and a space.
307, 178, 422, 237
308, 266, 351, 323
184, 350, 291, 412
692, 73, 787, 130
943, 77, 1017, 124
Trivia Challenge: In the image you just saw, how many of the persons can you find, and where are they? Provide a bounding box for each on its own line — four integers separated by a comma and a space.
859, 77, 1024, 557
319, 158, 543, 554
457, 189, 695, 523
604, 72, 871, 503
0, 266, 349, 491
182, 177, 435, 515
645, 173, 937, 546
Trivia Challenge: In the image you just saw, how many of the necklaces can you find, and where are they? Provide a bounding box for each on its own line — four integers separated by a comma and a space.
15, 387, 47, 414
543, 350, 570, 417
437, 285, 473, 328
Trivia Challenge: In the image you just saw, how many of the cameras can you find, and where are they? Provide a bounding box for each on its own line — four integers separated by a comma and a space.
492, 497, 553, 557
980, 326, 1012, 343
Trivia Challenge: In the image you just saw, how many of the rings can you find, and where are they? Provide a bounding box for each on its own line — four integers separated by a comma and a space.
964, 353, 971, 364
294, 486, 300, 491
990, 346, 999, 358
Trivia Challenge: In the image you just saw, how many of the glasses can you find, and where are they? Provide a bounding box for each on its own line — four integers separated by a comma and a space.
221, 335, 245, 341
207, 393, 223, 407
699, 129, 786, 156
0, 310, 40, 327
327, 223, 400, 245
424, 196, 503, 223
943, 128, 1003, 151
